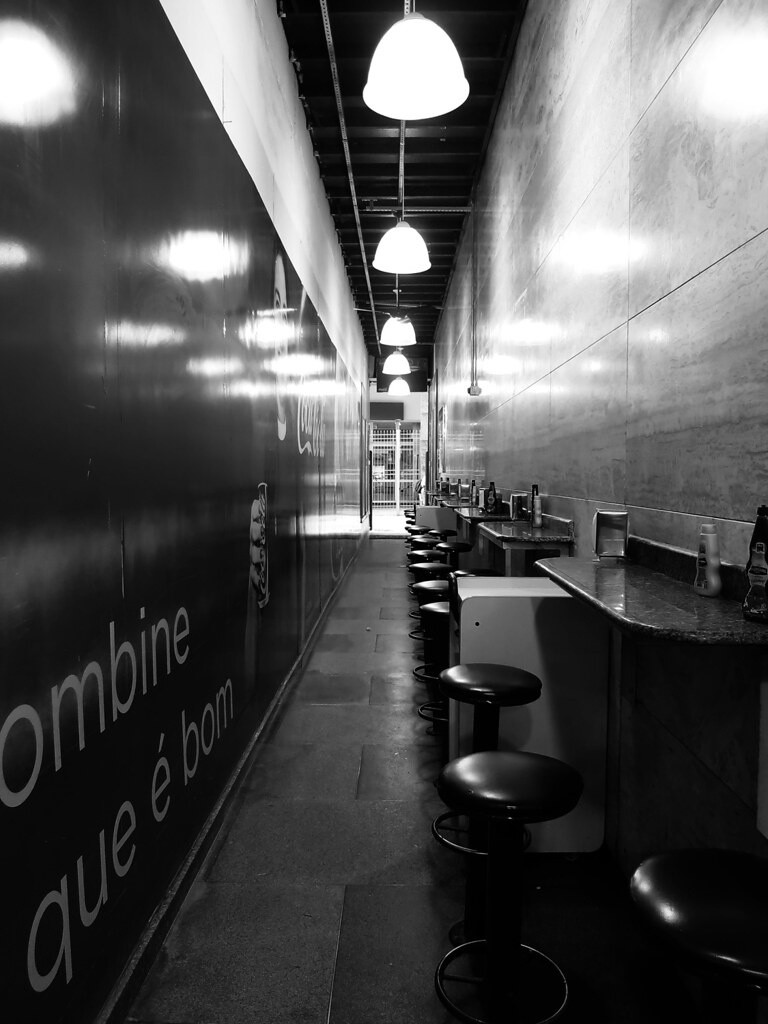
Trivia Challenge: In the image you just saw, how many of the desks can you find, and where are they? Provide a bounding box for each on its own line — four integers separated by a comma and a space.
426, 490, 575, 577
533, 554, 768, 872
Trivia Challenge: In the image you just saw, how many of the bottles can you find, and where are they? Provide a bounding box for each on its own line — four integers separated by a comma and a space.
440, 477, 497, 514
744, 505, 768, 623
532, 484, 543, 528
693, 525, 722, 595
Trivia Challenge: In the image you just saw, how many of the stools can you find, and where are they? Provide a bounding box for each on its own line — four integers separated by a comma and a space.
405, 524, 584, 1024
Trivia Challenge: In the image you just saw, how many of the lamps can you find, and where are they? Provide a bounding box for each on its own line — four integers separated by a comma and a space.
379, 308, 417, 392
362, 0, 472, 122
371, 211, 432, 275
